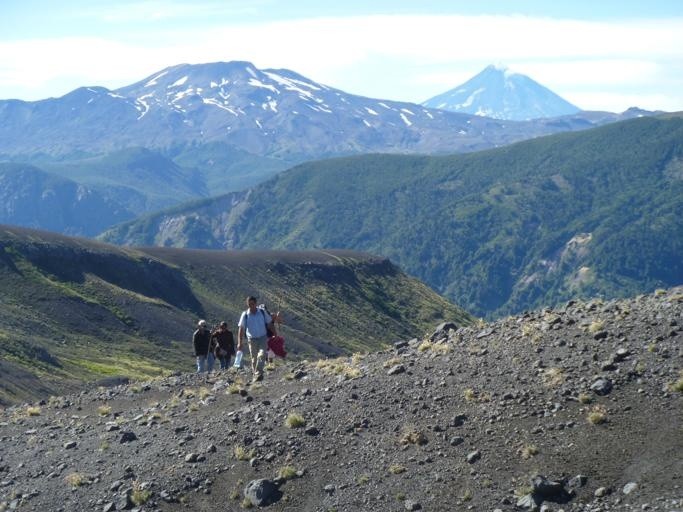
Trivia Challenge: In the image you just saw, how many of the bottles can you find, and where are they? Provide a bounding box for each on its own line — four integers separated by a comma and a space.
267, 348, 274, 368
233, 350, 243, 368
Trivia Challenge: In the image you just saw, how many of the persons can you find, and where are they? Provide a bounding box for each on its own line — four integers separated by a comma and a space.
237, 295, 277, 382
257, 303, 281, 368
192, 319, 215, 373
210, 321, 235, 373
